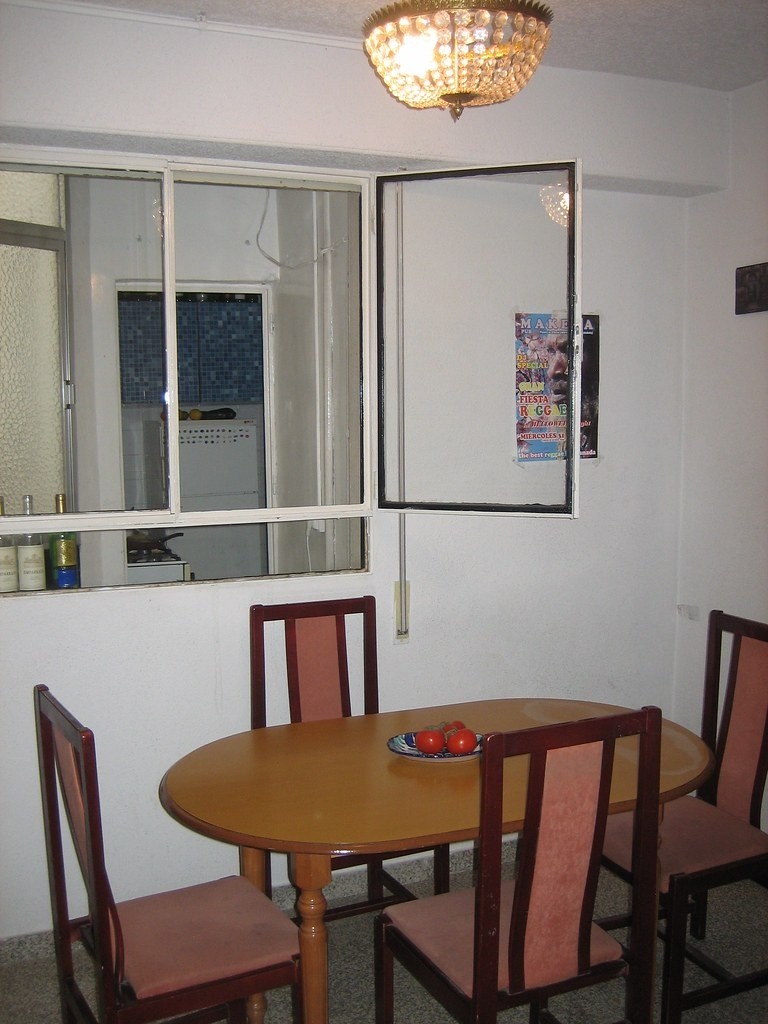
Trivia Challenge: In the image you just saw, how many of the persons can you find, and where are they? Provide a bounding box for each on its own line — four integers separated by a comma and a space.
544, 316, 600, 459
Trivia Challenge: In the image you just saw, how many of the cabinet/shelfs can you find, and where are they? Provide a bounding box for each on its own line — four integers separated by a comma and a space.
127, 560, 191, 583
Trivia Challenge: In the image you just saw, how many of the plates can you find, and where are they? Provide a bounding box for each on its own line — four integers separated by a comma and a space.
387, 732, 484, 763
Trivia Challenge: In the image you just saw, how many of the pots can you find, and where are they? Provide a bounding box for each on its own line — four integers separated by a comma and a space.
126, 533, 183, 550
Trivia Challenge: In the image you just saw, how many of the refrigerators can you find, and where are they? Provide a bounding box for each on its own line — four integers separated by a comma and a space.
161, 417, 265, 580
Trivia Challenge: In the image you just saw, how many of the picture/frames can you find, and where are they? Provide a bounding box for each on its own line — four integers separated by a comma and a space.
733, 261, 768, 316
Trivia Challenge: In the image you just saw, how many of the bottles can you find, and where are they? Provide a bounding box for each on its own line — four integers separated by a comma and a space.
0, 495, 19, 592
47, 494, 79, 589
17, 495, 46, 591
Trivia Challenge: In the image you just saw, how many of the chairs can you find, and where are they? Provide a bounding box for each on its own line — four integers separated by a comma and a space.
371, 703, 668, 1024
246, 593, 454, 928
32, 683, 299, 1024
599, 608, 768, 1024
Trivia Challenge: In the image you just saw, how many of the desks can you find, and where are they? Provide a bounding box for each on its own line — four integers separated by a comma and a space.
158, 693, 718, 1024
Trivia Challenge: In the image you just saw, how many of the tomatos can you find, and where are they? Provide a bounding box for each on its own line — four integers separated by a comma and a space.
416, 720, 477, 754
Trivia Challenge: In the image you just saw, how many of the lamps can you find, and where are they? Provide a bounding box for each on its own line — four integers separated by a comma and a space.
362, 0, 556, 121
538, 178, 570, 229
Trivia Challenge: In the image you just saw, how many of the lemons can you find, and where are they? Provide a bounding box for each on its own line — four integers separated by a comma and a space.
189, 409, 203, 420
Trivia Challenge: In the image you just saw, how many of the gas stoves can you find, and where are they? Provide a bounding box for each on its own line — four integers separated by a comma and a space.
126, 547, 195, 584
